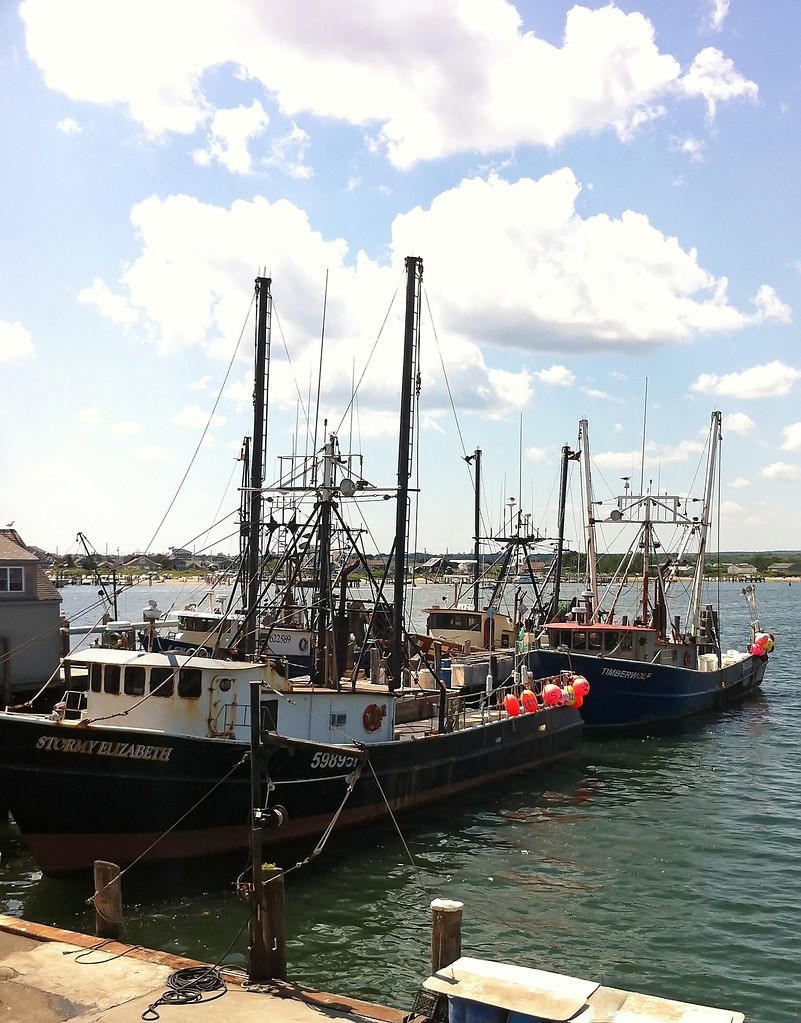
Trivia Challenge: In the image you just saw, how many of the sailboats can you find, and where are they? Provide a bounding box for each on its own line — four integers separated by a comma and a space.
393, 442, 555, 695
512, 409, 767, 729
131, 424, 357, 678
1, 254, 588, 874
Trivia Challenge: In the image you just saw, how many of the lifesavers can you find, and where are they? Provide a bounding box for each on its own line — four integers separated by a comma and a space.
299, 638, 308, 650
684, 651, 692, 665
363, 704, 382, 731
